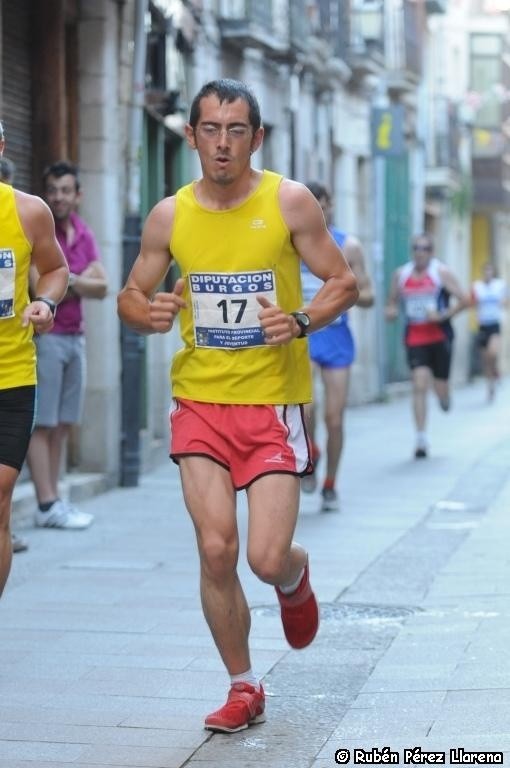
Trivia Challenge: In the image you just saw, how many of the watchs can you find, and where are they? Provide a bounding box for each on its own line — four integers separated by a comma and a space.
35, 296, 58, 321
68, 272, 76, 292
290, 311, 310, 338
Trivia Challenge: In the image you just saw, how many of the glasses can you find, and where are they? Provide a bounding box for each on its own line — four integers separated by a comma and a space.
199, 124, 248, 140
411, 245, 431, 251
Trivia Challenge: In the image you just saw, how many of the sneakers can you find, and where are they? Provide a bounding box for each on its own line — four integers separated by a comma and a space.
34, 501, 92, 531
301, 445, 320, 493
10, 532, 28, 554
61, 497, 94, 522
415, 440, 427, 458
320, 485, 340, 512
273, 548, 320, 650
203, 679, 268, 734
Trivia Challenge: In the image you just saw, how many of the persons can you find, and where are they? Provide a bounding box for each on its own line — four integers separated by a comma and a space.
466, 260, 510, 407
300, 183, 375, 513
29, 162, 107, 530
116, 79, 360, 734
384, 237, 469, 459
0, 125, 69, 620
